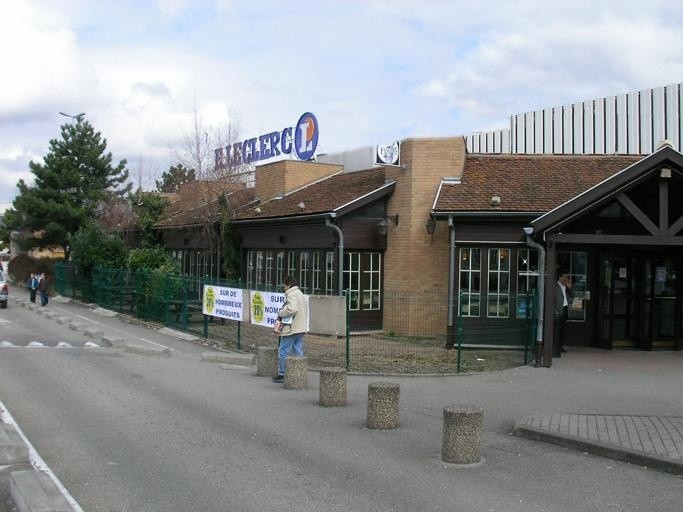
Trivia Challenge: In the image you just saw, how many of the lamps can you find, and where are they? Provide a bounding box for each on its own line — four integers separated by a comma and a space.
378, 214, 399, 237
426, 216, 436, 235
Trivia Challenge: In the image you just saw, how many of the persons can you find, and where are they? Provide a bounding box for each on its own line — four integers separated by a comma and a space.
38, 272, 49, 306
556, 272, 576, 352
271, 276, 310, 382
28, 272, 39, 303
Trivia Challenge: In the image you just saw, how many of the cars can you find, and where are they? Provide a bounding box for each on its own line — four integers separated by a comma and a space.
0, 268, 8, 308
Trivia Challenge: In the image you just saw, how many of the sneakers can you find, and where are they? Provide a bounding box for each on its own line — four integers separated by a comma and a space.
272, 375, 284, 383
